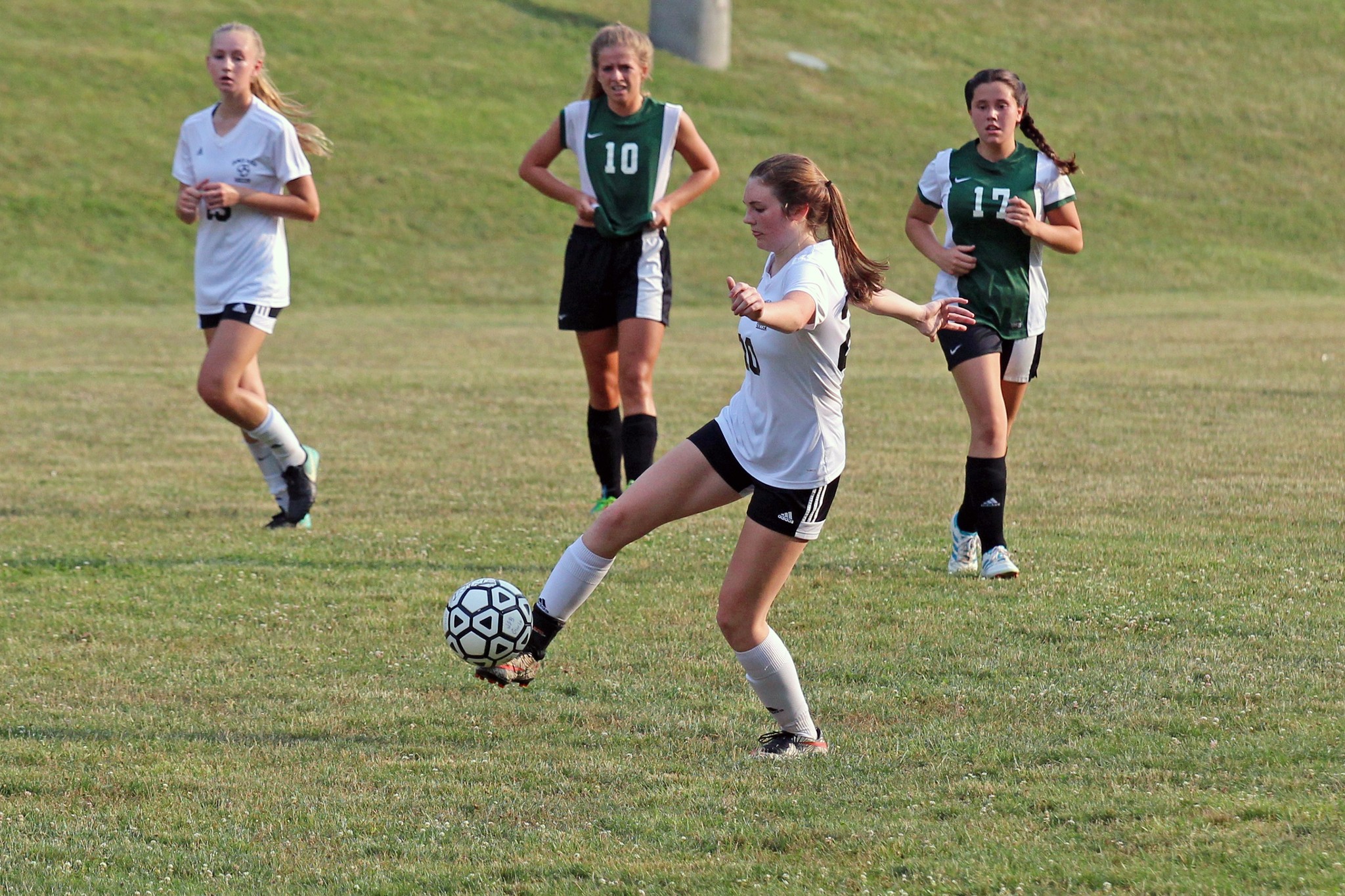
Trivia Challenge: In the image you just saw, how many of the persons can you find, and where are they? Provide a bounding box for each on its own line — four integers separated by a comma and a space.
171, 21, 335, 531
469, 153, 979, 758
516, 21, 721, 513
904, 67, 1084, 584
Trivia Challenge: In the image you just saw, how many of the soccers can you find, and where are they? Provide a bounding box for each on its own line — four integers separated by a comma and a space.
442, 577, 534, 668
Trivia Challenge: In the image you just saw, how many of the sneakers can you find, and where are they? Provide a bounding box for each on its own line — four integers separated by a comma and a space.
590, 496, 617, 512
981, 545, 1019, 579
947, 511, 981, 573
625, 479, 635, 489
759, 727, 827, 760
471, 645, 546, 688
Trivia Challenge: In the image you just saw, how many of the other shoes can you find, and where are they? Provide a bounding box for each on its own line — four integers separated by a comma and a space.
281, 444, 321, 524
264, 509, 312, 529
